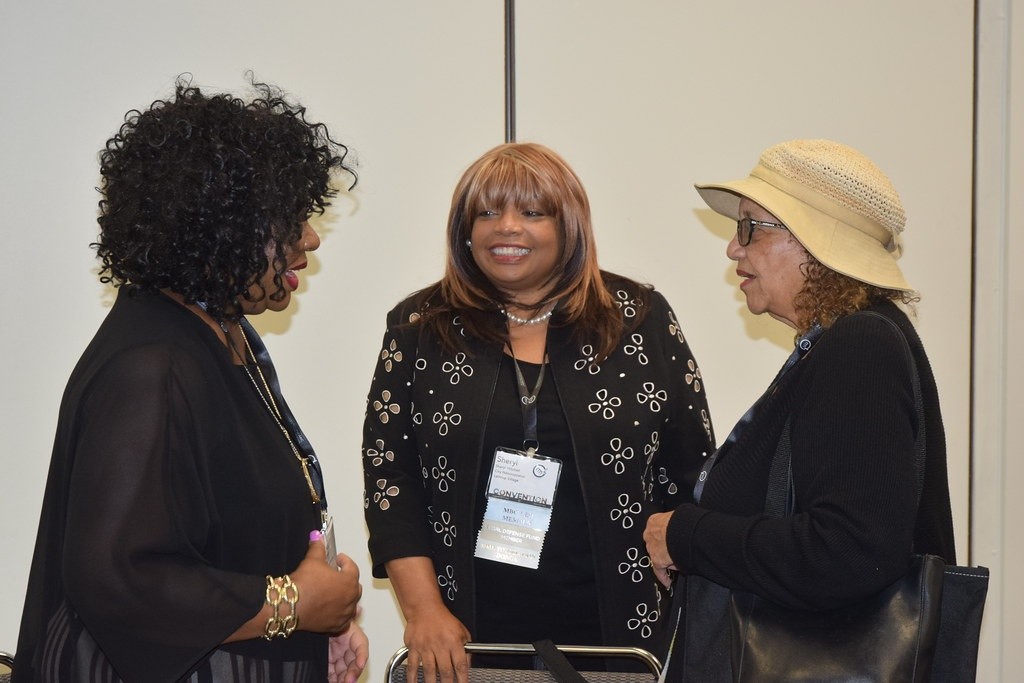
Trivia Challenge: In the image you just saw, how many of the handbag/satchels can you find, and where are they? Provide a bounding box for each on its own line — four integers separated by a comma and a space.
680, 310, 989, 683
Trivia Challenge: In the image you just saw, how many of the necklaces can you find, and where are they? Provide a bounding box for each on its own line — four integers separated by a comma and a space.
238, 323, 322, 504
498, 305, 553, 324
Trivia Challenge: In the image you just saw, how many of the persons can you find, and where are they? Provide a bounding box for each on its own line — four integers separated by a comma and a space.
10, 76, 371, 683
643, 138, 958, 683
360, 142, 716, 683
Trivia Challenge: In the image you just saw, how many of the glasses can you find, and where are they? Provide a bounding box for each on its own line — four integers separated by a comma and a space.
737, 217, 788, 247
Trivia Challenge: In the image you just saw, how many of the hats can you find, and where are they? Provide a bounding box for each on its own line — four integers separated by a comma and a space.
693, 137, 915, 293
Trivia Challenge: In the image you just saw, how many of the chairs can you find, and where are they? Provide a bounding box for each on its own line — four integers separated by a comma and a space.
383, 642, 665, 683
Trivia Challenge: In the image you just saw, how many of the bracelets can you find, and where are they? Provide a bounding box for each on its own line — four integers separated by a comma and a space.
262, 574, 300, 642
